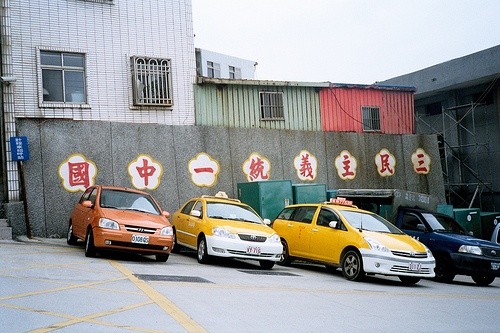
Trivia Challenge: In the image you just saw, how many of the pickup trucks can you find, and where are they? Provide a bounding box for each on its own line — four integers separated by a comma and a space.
393, 205, 500, 285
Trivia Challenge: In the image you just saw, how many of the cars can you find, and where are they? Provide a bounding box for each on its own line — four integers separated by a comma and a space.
67, 185, 174, 262
270, 197, 436, 285
171, 191, 283, 269
